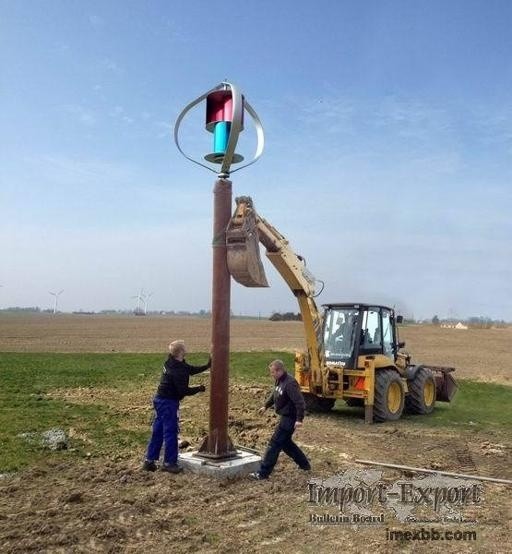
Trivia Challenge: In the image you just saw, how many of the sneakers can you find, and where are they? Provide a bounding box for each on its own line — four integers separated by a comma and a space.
144, 460, 183, 474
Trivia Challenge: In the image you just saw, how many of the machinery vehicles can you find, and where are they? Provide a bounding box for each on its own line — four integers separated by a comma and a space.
226, 192, 462, 423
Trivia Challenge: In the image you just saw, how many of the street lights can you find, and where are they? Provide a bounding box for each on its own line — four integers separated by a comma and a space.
172, 77, 265, 477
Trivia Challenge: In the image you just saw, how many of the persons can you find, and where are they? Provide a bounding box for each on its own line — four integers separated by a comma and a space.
143, 339, 212, 474
247, 359, 312, 481
333, 315, 364, 355
